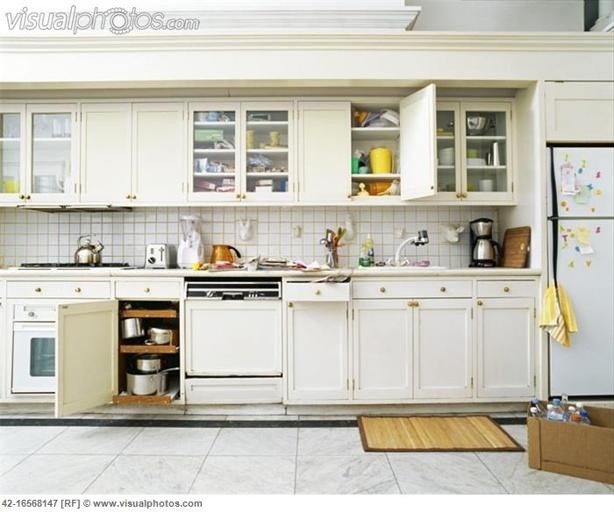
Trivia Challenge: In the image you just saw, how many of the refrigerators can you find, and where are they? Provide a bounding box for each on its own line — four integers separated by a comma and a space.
546, 145, 614, 399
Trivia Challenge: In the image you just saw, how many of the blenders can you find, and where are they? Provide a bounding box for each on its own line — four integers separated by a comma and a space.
174, 214, 205, 270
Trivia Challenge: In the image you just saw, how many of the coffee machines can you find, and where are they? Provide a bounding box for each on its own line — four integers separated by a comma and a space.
467, 218, 503, 268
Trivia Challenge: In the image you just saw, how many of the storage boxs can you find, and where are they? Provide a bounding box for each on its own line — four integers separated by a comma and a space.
525, 401, 614, 488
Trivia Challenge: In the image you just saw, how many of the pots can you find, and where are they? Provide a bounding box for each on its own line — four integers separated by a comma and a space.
467, 116, 495, 136
120, 317, 144, 341
124, 355, 178, 396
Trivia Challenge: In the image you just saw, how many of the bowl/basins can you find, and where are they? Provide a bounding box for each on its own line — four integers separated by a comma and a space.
33, 176, 63, 194
439, 148, 453, 166
467, 158, 485, 166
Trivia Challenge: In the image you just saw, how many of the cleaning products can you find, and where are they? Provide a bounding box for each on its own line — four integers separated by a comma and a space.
365, 233, 375, 266
359, 240, 370, 267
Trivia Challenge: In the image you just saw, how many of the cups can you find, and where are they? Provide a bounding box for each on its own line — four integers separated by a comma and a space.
324, 249, 337, 269
467, 148, 477, 158
246, 130, 254, 150
479, 179, 492, 191
268, 131, 280, 147
5, 179, 19, 193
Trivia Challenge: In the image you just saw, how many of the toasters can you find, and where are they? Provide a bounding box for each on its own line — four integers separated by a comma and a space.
144, 243, 170, 270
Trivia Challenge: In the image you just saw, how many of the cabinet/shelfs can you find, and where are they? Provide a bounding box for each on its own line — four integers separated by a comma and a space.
112, 278, 186, 417
407, 97, 515, 207
475, 275, 542, 415
351, 274, 478, 418
0, 97, 81, 208
279, 273, 351, 419
5, 275, 112, 304
299, 83, 439, 207
189, 97, 297, 208
545, 80, 613, 145
81, 98, 188, 208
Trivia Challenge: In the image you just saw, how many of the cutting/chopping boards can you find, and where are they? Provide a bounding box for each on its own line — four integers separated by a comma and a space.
499, 226, 531, 268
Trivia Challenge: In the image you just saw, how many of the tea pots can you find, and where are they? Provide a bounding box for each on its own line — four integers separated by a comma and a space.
210, 244, 241, 264
74, 234, 103, 265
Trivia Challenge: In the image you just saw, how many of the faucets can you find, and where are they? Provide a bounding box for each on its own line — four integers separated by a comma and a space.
395, 230, 428, 267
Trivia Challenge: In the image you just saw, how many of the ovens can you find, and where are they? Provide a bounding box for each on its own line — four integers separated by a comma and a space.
182, 278, 282, 408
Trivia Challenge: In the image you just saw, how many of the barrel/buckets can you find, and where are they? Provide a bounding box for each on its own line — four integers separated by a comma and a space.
34, 174, 64, 193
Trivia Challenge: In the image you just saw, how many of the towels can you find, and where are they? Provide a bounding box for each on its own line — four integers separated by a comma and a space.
537, 283, 580, 349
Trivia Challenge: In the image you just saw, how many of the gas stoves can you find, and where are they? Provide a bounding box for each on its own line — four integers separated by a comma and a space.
17, 261, 127, 268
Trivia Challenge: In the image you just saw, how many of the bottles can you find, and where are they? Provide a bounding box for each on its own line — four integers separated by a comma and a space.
467, 172, 475, 191
368, 182, 391, 196
487, 151, 493, 166
489, 141, 499, 166
528, 392, 590, 426
357, 234, 375, 267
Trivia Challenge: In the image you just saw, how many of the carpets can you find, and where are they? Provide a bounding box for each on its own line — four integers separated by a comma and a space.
356, 412, 527, 454
